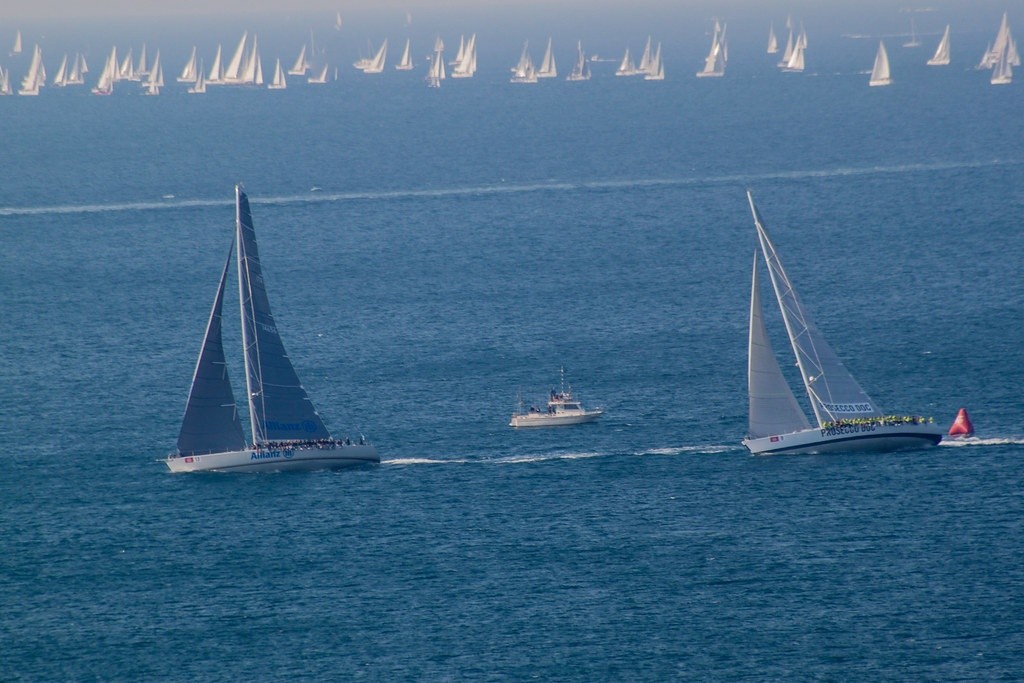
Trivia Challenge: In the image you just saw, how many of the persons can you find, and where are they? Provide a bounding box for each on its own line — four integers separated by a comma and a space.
251, 438, 364, 450
167, 452, 176, 460
822, 415, 934, 428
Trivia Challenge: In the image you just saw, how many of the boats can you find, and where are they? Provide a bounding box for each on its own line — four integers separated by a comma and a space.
508, 365, 605, 427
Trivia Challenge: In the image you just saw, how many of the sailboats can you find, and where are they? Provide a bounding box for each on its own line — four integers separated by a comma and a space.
0, 25, 667, 100
975, 12, 1020, 85
740, 187, 944, 458
764, 15, 810, 73
923, 26, 952, 67
153, 179, 383, 474
867, 37, 893, 87
694, 20, 730, 78
